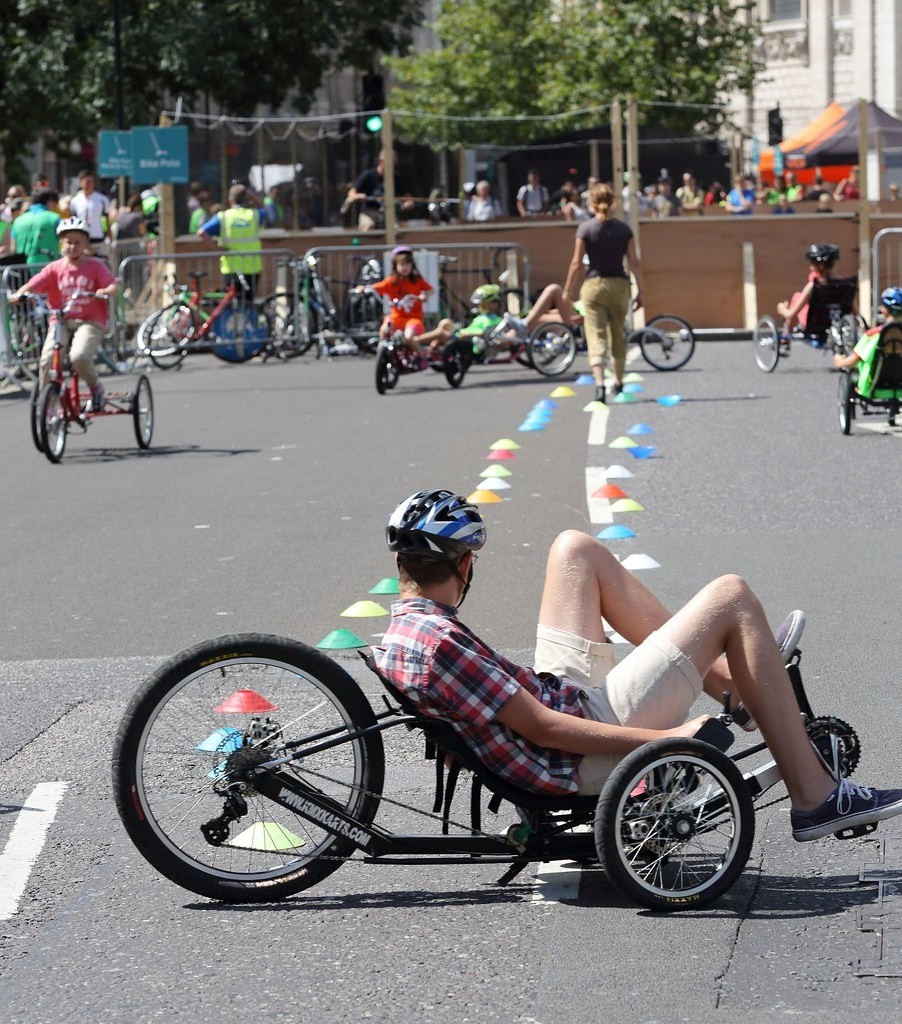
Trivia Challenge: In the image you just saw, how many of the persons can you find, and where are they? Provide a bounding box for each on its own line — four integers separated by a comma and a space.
195, 184, 269, 304
562, 183, 644, 403
340, 148, 902, 232
761, 243, 861, 349
354, 246, 434, 369
369, 488, 902, 841
8, 218, 119, 415
0, 169, 325, 379
832, 287, 902, 394
413, 285, 633, 360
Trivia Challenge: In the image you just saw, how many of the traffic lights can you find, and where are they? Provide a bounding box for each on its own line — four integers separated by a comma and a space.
362, 75, 385, 134
769, 110, 783, 145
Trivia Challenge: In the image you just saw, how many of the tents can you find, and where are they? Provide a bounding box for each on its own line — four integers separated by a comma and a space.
750, 99, 902, 187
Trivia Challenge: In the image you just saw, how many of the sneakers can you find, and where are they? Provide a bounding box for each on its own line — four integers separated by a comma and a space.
790, 772, 902, 842
731, 609, 805, 733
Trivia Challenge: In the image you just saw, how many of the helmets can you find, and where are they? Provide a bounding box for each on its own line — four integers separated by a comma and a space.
881, 286, 902, 310
57, 216, 90, 237
806, 242, 840, 265
386, 489, 486, 561
582, 253, 590, 265
470, 283, 501, 305
393, 245, 413, 258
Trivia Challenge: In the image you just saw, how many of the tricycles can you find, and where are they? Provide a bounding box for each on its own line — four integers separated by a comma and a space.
110, 629, 881, 912
470, 245, 694, 377
348, 288, 469, 395
752, 277, 902, 435
18, 290, 152, 464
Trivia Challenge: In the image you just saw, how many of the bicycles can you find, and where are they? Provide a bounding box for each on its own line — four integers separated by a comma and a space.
135, 256, 386, 370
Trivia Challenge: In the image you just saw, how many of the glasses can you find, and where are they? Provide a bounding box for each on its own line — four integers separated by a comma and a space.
462, 549, 480, 565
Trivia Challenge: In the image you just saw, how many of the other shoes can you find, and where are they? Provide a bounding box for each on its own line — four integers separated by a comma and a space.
92, 388, 106, 414
611, 383, 623, 394
396, 330, 408, 348
503, 312, 529, 338
595, 385, 605, 403
